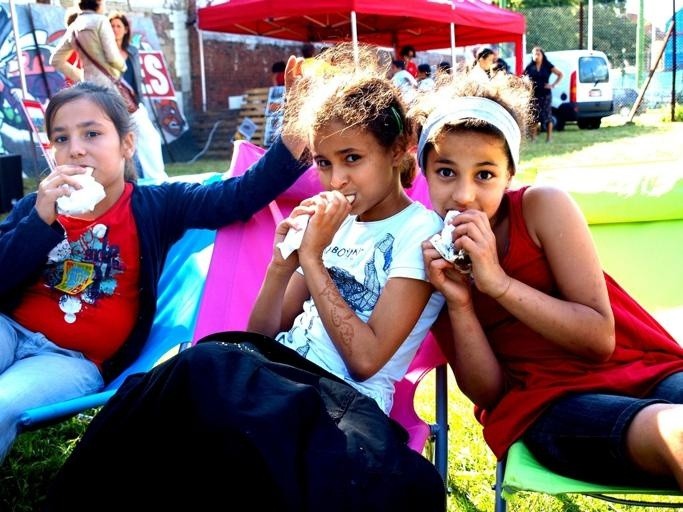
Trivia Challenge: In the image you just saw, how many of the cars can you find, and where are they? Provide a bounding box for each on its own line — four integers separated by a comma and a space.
606, 84, 647, 121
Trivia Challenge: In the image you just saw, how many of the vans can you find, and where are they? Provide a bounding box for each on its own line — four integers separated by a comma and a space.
493, 47, 616, 133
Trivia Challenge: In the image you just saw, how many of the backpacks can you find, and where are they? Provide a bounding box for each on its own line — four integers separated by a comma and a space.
35, 331, 446, 509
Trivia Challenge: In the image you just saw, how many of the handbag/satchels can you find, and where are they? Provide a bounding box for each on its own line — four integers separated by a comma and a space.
72, 27, 137, 113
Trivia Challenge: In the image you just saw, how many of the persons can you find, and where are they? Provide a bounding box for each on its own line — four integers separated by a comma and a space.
268, 42, 565, 145
0, 53, 316, 467
106, 13, 168, 181
46, 0, 143, 181
416, 65, 683, 497
61, 13, 84, 87
246, 73, 453, 416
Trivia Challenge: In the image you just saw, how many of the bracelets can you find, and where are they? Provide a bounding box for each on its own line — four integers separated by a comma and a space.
492, 275, 512, 300
